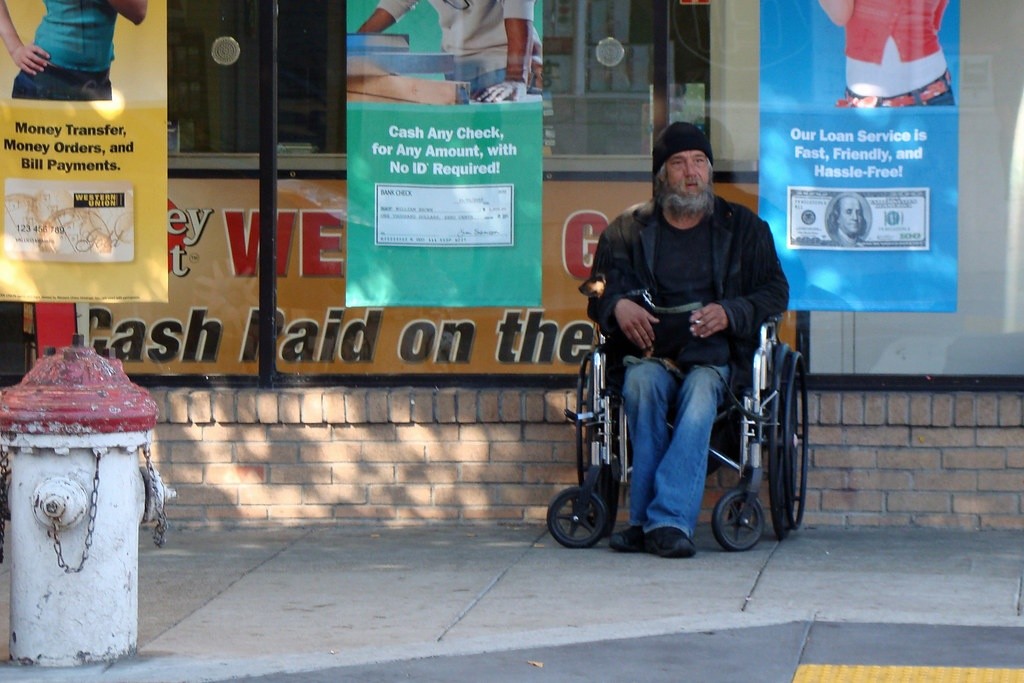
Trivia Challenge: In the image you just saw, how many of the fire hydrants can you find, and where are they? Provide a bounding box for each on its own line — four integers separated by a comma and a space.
0, 337, 180, 667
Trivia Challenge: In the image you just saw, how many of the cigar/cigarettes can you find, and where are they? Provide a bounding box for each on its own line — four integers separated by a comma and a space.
694, 319, 701, 324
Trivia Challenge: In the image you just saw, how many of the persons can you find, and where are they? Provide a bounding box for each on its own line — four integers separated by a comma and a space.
588, 120, 791, 560
0, 0, 147, 101
357, 0, 541, 104
820, 0, 955, 109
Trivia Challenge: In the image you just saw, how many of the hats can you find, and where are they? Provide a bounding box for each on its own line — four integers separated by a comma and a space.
652, 121, 714, 176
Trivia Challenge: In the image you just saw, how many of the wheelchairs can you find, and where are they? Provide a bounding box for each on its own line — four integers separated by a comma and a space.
547, 284, 811, 548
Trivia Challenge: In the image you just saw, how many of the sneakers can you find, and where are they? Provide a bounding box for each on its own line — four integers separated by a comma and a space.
609, 526, 696, 557
